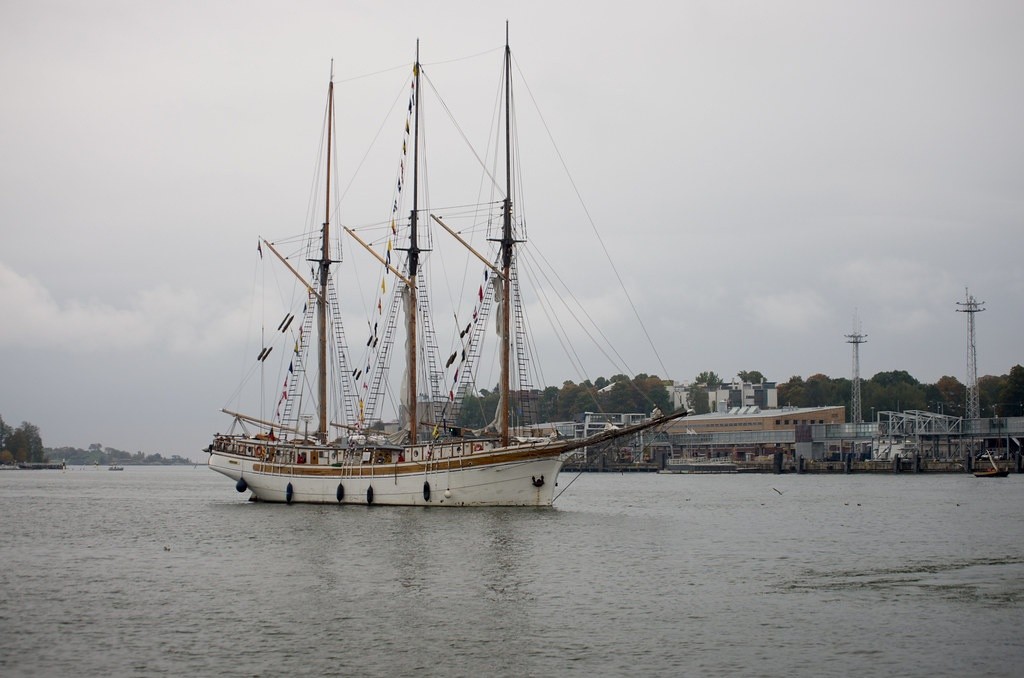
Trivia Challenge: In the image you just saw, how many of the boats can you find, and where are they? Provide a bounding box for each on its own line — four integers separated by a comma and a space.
19, 462, 64, 471
659, 458, 740, 475
108, 467, 124, 472
974, 468, 1010, 477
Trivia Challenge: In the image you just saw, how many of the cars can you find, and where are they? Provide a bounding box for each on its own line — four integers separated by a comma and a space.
994, 453, 1008, 460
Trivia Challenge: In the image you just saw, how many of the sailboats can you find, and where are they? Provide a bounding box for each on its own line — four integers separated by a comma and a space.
201, 21, 689, 511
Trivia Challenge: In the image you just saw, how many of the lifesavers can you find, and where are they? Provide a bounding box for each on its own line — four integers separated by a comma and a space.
473, 443, 483, 451
238, 445, 245, 452
255, 445, 263, 456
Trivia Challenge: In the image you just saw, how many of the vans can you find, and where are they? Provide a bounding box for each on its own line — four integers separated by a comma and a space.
981, 451, 997, 461
